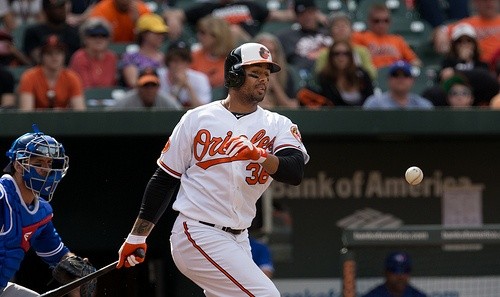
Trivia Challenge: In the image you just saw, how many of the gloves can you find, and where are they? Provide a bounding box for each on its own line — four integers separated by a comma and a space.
224, 137, 268, 165
116, 233, 148, 269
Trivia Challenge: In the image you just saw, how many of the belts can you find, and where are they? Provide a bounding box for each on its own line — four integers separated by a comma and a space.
199, 221, 245, 235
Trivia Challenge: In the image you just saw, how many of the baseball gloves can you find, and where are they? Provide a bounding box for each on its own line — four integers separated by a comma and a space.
53, 256, 97, 297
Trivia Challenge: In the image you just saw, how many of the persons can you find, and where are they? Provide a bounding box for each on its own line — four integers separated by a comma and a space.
0, 0, 500, 111
119, 43, 309, 297
364, 249, 430, 297
0, 123, 97, 297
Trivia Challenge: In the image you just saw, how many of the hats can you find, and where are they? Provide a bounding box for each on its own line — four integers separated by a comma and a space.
165, 40, 192, 65
452, 23, 476, 40
134, 13, 169, 35
40, 34, 66, 58
137, 66, 161, 86
390, 61, 411, 76
3, 132, 62, 174
386, 250, 411, 271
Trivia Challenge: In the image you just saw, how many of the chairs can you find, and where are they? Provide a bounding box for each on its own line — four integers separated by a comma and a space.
8, 0, 453, 109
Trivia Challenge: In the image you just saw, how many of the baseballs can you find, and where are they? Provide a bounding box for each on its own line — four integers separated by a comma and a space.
405, 167, 423, 185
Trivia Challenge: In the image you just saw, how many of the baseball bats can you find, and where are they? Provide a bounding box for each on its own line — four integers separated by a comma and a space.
37, 247, 145, 297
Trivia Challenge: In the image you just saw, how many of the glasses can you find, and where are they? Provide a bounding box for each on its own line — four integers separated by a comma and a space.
331, 50, 350, 58
371, 17, 389, 24
391, 71, 409, 77
86, 28, 108, 38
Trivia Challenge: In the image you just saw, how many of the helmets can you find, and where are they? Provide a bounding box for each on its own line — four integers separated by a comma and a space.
224, 42, 281, 88
